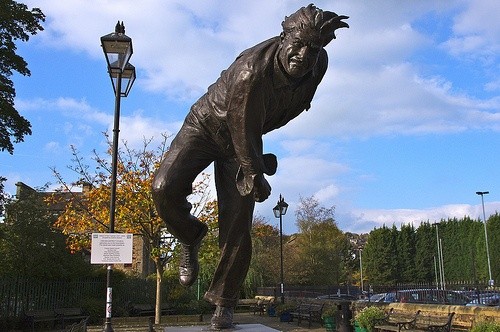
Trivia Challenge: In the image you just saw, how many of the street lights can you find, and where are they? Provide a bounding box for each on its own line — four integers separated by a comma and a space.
272, 194, 289, 304
358, 246, 363, 292
475, 191, 492, 288
100, 20, 137, 330
431, 223, 446, 291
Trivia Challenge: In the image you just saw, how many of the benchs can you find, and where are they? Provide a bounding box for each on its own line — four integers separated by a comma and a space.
371, 307, 420, 332
249, 297, 270, 316
234, 299, 255, 312
128, 302, 175, 315
24, 307, 87, 332
289, 302, 324, 328
397, 310, 455, 332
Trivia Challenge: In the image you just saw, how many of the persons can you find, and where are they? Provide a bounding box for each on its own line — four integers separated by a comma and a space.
152, 3, 350, 328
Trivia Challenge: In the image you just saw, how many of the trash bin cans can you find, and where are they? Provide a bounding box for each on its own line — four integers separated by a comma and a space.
334, 301, 353, 332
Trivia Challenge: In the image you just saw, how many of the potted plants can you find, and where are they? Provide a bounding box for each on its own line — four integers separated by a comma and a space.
349, 305, 388, 332
320, 304, 337, 332
274, 303, 296, 322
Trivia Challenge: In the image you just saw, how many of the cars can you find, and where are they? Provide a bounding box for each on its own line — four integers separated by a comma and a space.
313, 289, 500, 306
